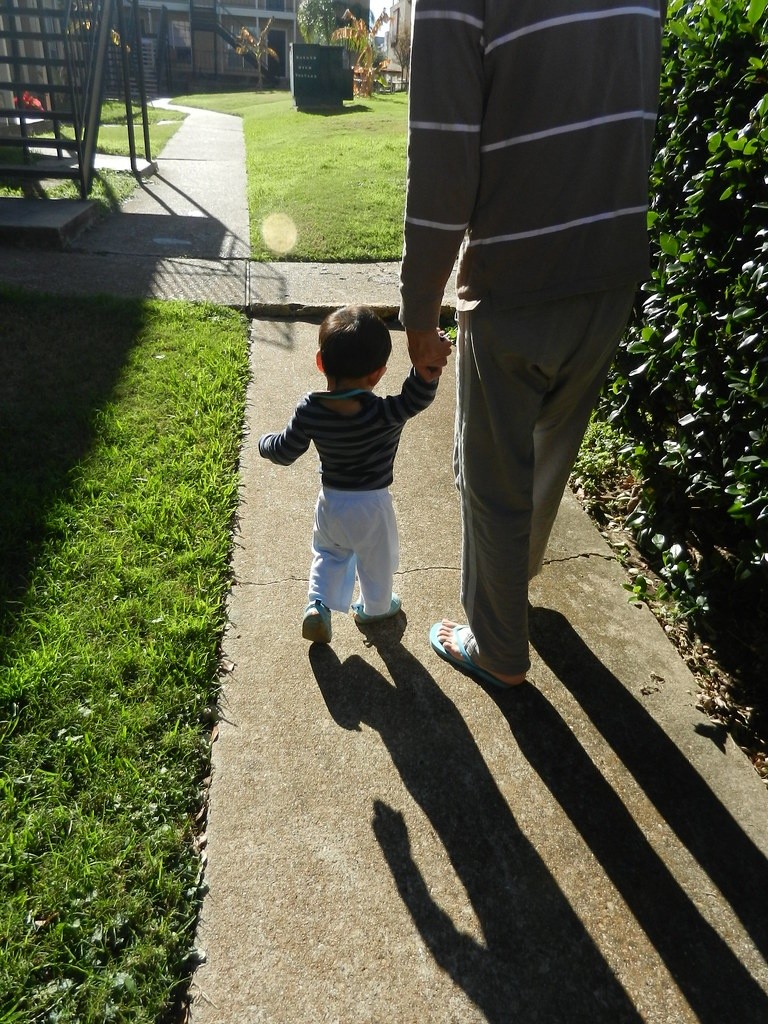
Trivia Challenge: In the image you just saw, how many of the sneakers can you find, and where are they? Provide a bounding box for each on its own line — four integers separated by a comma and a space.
351, 593, 402, 624
301, 599, 332, 643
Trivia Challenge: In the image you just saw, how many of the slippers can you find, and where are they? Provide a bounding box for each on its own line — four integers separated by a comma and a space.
430, 622, 512, 690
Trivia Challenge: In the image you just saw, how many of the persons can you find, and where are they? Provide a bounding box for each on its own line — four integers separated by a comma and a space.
397, 0, 668, 690
258, 304, 447, 643
